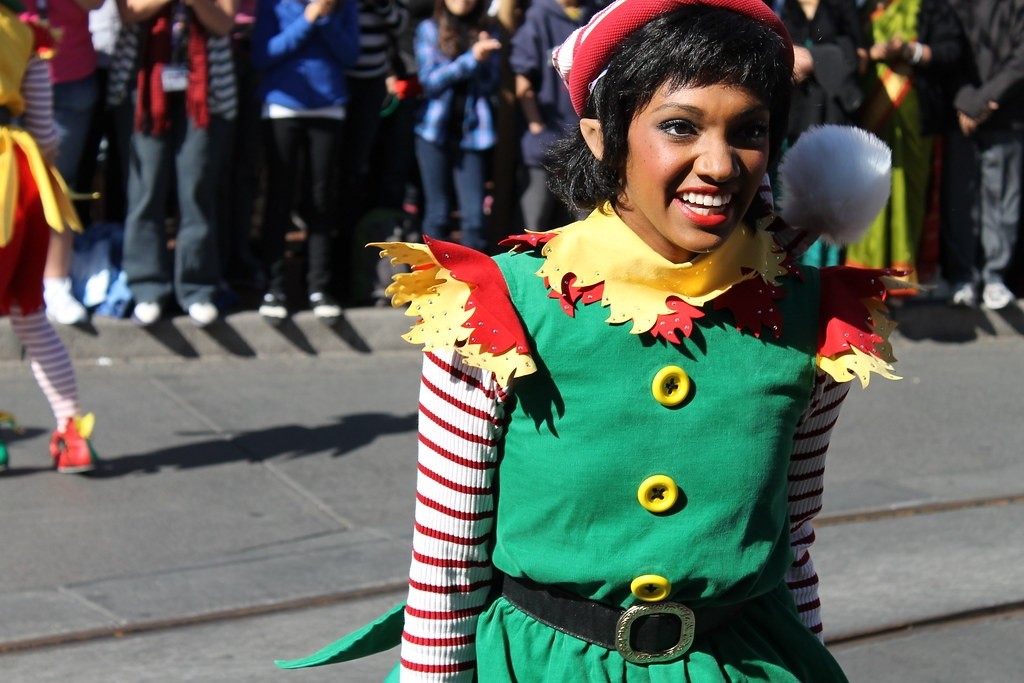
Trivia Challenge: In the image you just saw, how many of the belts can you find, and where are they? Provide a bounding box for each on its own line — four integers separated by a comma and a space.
493, 569, 791, 667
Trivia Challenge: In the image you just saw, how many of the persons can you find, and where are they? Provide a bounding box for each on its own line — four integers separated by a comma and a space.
401, 0, 920, 683
0, 0, 609, 472
768, 0, 1024, 310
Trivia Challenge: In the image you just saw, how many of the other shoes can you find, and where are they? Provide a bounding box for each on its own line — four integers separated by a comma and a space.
133, 300, 162, 327
45, 278, 88, 325
260, 294, 288, 325
49, 414, 98, 474
311, 296, 342, 325
189, 303, 219, 328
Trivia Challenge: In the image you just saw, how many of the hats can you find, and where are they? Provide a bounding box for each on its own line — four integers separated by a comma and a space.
549, 0, 795, 117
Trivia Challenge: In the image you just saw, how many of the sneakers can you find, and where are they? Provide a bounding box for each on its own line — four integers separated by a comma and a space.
951, 282, 974, 309
983, 281, 1013, 310
